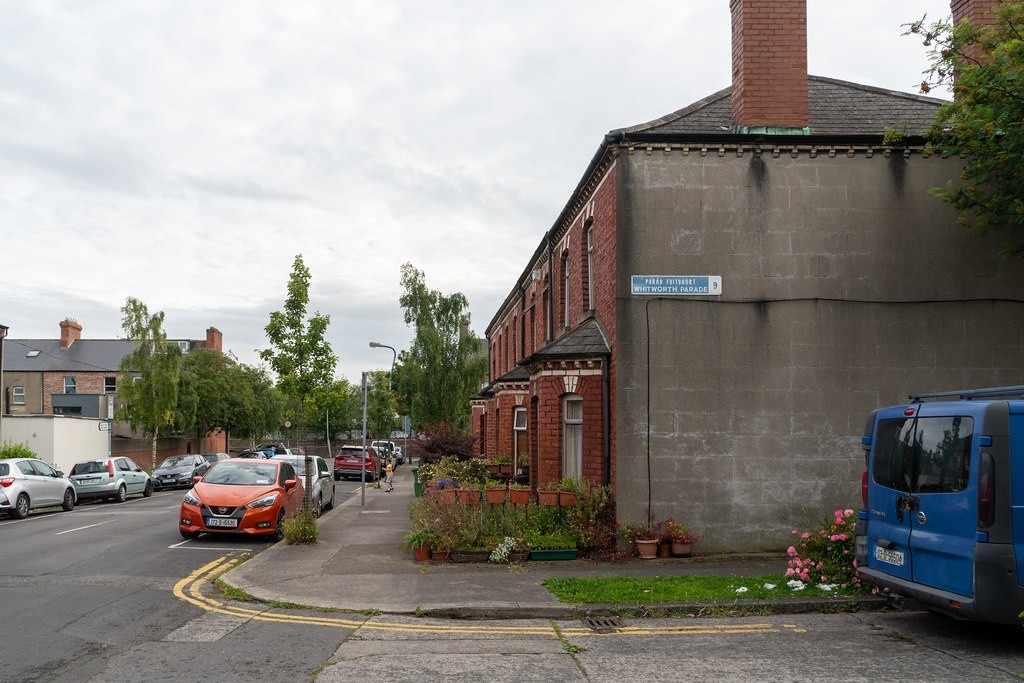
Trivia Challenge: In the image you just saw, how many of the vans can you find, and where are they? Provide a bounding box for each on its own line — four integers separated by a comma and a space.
856, 384, 1022, 634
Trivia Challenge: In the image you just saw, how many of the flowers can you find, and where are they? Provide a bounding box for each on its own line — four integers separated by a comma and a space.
417, 455, 489, 483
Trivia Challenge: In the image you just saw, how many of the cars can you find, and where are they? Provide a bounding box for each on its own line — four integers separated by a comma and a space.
0, 457, 78, 520
179, 458, 308, 541
69, 456, 153, 501
269, 454, 336, 519
151, 454, 210, 491
334, 444, 380, 482
200, 443, 304, 465
372, 439, 405, 472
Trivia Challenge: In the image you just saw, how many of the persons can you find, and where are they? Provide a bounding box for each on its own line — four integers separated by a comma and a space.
383, 458, 394, 493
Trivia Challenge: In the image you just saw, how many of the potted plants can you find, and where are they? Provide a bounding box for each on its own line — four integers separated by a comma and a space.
402, 450, 701, 564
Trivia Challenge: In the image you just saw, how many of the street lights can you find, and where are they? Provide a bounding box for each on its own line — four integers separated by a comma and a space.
369, 342, 397, 393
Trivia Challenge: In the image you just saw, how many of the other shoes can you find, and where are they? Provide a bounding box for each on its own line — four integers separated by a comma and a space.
390, 488, 393, 490
385, 489, 390, 492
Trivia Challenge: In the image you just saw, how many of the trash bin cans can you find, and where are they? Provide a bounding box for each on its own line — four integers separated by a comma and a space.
412, 468, 432, 497
